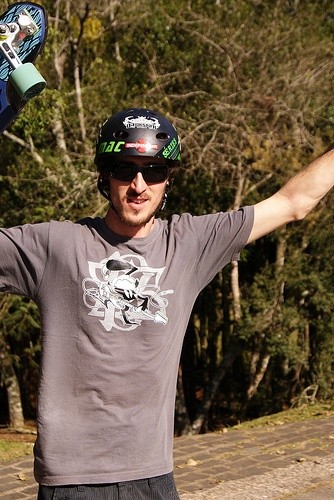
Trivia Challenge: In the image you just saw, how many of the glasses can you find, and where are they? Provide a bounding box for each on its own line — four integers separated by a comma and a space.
104, 165, 175, 184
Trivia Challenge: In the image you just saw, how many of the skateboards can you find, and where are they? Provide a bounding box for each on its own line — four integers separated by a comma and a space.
0, 1, 48, 136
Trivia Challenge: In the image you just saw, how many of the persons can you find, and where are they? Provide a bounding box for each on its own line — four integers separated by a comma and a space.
0, 110, 334, 500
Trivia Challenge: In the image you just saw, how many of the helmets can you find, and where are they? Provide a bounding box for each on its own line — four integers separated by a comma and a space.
94, 108, 194, 163
